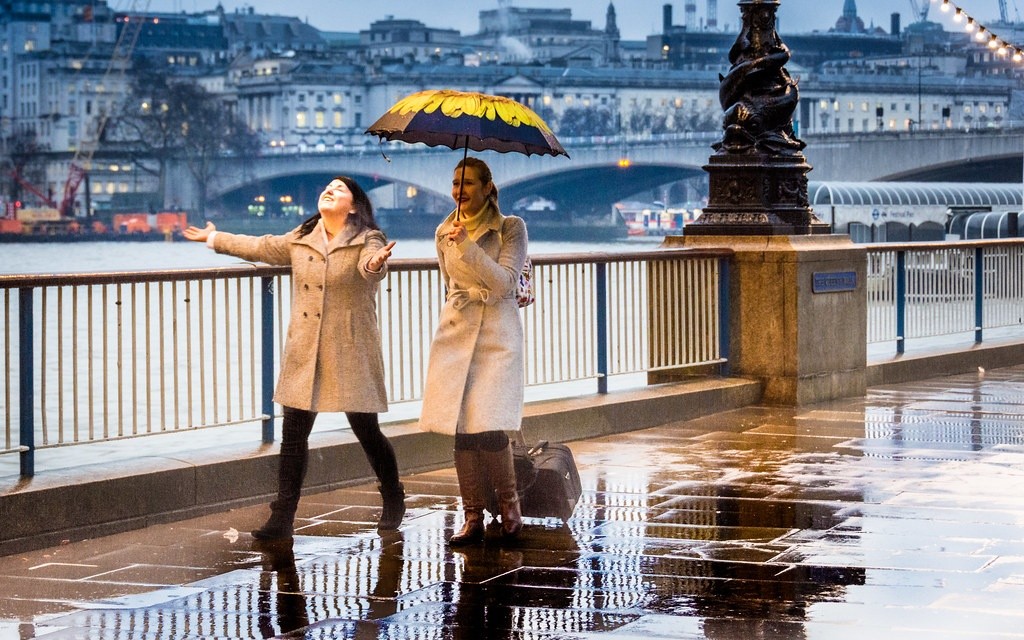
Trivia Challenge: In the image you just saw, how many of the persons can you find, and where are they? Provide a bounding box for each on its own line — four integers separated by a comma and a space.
183, 176, 405, 537
418, 157, 527, 542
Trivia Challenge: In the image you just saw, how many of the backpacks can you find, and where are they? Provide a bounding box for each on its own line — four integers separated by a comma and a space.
497, 215, 534, 308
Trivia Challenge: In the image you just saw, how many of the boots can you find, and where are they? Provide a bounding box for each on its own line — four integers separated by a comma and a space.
446, 545, 485, 607
495, 544, 522, 606
376, 532, 407, 557
377, 483, 406, 530
479, 434, 522, 537
251, 540, 294, 556
449, 449, 484, 545
251, 501, 298, 539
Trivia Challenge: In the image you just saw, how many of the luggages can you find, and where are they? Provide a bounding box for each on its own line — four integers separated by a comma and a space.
487, 426, 582, 522
460, 524, 582, 584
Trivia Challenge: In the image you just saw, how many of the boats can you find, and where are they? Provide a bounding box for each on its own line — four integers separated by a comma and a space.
808, 181, 1024, 302
613, 201, 709, 236
0, 206, 196, 243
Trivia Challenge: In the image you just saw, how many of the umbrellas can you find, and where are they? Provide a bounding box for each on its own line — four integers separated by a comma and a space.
364, 89, 570, 246
